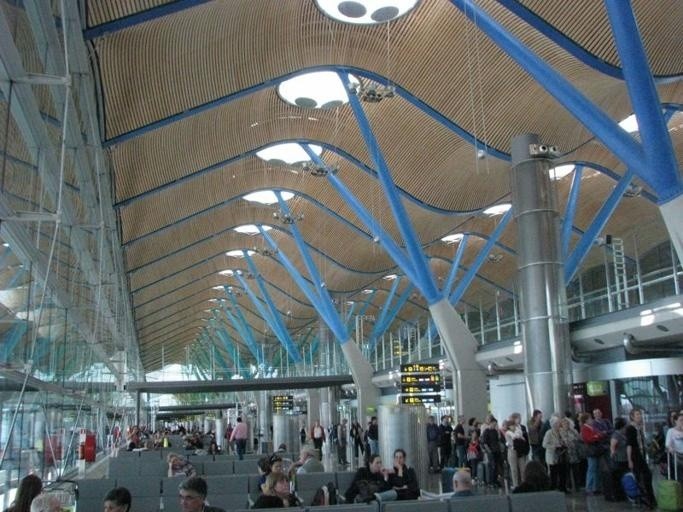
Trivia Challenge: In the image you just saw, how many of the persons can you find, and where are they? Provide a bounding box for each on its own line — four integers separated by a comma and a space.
2, 405, 683, 511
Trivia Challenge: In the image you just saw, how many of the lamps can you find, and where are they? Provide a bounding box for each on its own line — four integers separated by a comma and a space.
618, 113, 638, 134
344, 203, 512, 306
255, 143, 339, 178
312, 0, 418, 24
278, 71, 393, 109
196, 225, 276, 335
243, 191, 303, 224
549, 164, 576, 182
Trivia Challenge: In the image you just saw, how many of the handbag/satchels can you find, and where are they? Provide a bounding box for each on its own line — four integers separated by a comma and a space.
572, 439, 606, 459
601, 470, 625, 502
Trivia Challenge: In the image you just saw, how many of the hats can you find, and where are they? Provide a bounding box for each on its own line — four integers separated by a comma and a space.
177, 476, 208, 490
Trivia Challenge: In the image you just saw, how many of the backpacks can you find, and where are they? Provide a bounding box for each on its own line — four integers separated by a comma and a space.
621, 469, 646, 504
311, 481, 337, 506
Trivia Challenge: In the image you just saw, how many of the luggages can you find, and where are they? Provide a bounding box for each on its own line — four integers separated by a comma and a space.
656, 449, 682, 510
477, 460, 489, 486
